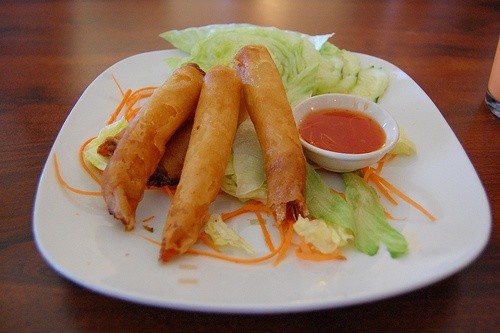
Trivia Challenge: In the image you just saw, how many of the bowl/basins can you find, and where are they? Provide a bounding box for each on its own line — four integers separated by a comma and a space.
293, 94, 399, 174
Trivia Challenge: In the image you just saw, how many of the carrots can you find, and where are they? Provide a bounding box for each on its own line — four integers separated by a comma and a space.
53, 74, 436, 268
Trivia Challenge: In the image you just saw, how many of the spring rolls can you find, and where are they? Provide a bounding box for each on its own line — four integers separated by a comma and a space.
97, 44, 308, 263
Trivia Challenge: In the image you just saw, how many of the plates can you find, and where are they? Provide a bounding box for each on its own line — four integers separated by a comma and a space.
33, 44, 489, 318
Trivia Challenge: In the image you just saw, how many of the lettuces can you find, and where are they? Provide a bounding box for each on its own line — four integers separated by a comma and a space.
159, 23, 409, 259
